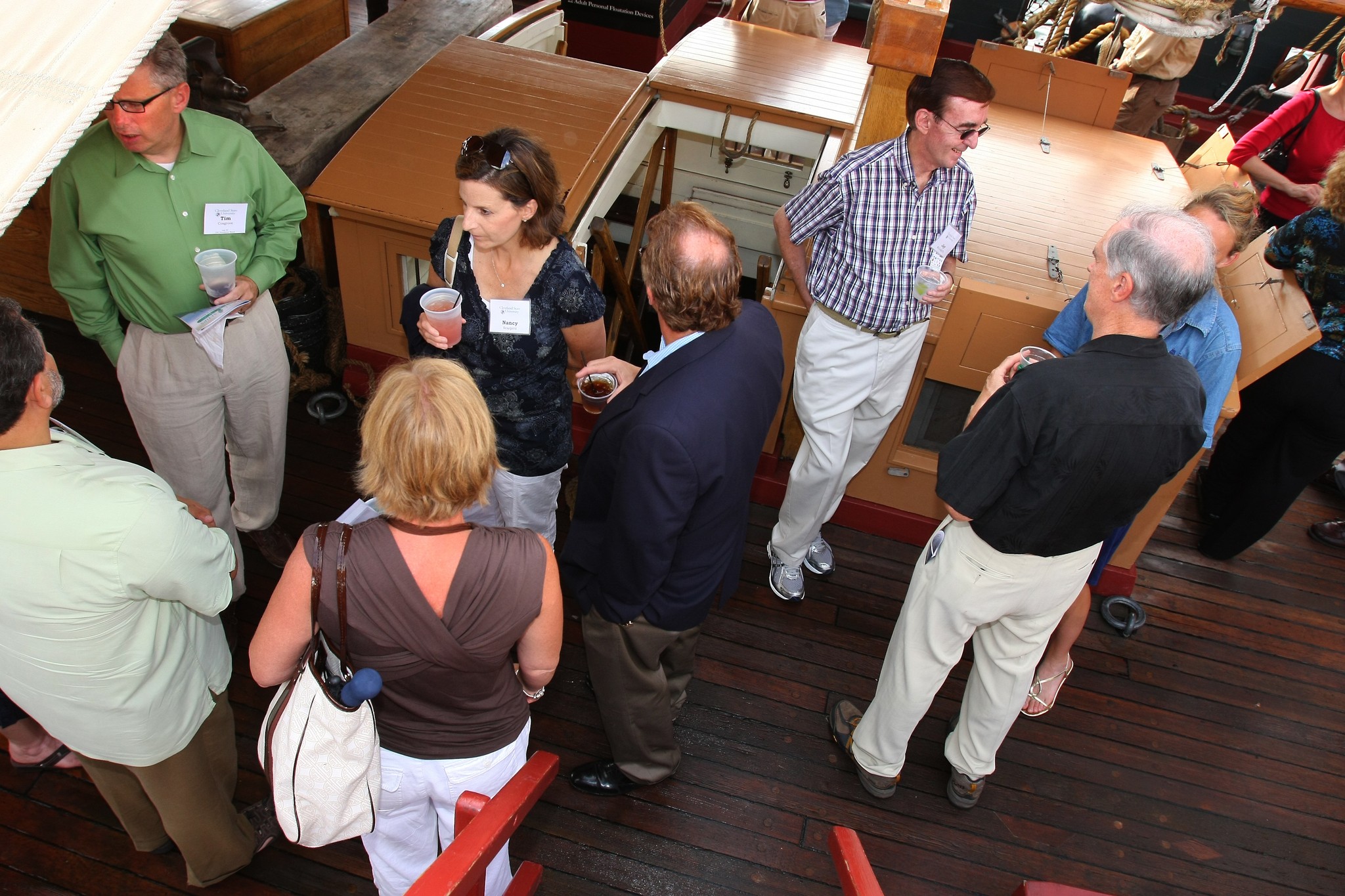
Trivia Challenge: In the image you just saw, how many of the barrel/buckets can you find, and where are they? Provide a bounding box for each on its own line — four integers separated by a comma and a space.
1143, 104, 1192, 162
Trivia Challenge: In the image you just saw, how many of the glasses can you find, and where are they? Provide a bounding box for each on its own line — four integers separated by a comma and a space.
928, 110, 990, 140
102, 87, 175, 114
461, 135, 521, 171
638, 244, 648, 258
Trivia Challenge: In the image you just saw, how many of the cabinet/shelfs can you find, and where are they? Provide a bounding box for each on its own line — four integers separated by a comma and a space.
0, 0, 1321, 615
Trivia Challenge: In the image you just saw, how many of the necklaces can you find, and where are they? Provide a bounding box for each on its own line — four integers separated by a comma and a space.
491, 247, 535, 288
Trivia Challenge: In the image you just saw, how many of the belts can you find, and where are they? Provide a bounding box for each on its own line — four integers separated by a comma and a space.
1137, 74, 1177, 82
816, 302, 929, 339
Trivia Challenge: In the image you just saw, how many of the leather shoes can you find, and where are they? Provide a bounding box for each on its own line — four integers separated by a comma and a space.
1308, 517, 1345, 550
1317, 466, 1339, 491
569, 759, 645, 797
245, 521, 295, 568
219, 602, 239, 656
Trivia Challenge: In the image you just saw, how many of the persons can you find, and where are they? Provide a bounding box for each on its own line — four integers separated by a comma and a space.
829, 200, 1215, 809
767, 56, 996, 606
416, 125, 607, 553
0, 297, 281, 896
723, 0, 849, 161
248, 356, 565, 896
1018, 182, 1261, 717
564, 196, 785, 797
47, 31, 307, 655
1227, 37, 1345, 233
1109, 22, 1204, 137
1196, 148, 1345, 559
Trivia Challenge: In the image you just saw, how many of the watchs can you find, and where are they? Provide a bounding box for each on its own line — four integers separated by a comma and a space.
944, 271, 955, 292
1111, 62, 1118, 69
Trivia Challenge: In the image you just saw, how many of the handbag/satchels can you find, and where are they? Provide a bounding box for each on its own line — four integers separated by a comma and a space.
257, 522, 380, 849
1251, 89, 1320, 191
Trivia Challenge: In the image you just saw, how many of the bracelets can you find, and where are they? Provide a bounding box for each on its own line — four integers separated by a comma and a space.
619, 621, 634, 627
514, 670, 545, 700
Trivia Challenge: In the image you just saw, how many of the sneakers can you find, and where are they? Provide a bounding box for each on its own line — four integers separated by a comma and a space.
767, 540, 805, 602
947, 712, 985, 809
829, 699, 897, 799
148, 839, 175, 855
240, 797, 283, 855
803, 530, 835, 575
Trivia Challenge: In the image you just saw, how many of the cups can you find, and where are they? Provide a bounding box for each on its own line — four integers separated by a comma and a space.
577, 372, 618, 414
194, 249, 238, 303
1020, 346, 1056, 367
419, 286, 463, 348
914, 265, 947, 301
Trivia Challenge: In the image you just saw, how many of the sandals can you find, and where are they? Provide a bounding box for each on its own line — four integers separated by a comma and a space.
1021, 651, 1074, 717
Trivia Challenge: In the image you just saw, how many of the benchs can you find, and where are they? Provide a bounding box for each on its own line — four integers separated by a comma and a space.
236, 0, 514, 273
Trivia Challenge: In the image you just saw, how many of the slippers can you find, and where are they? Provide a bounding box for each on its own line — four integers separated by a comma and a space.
9, 743, 96, 786
